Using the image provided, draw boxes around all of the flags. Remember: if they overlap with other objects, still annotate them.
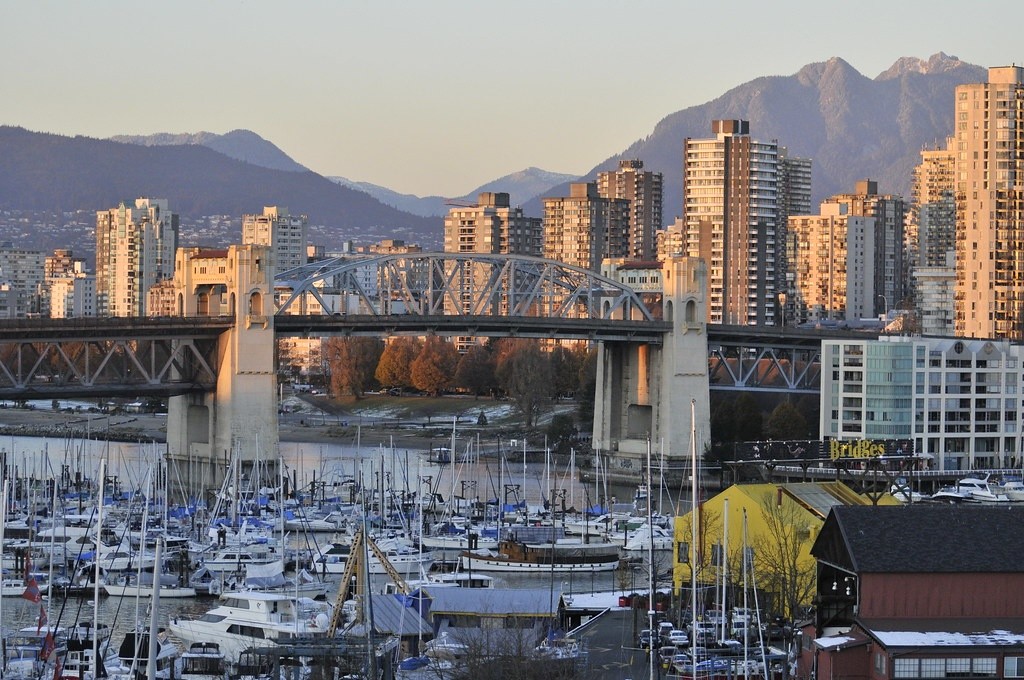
[22,578,41,603]
[22,554,32,586]
[40,631,55,660]
[37,605,48,636]
[53,657,62,680]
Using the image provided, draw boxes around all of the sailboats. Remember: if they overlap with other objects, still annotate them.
[0,413,776,680]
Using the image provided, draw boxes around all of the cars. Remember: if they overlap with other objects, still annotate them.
[633,605,755,667]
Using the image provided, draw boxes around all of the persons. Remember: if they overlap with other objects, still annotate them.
[750,437,807,460]
